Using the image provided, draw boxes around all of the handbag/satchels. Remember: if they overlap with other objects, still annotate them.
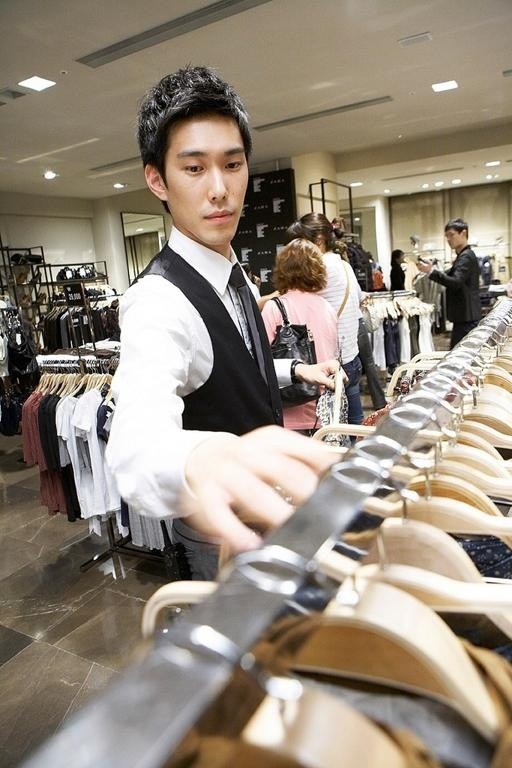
[270,296,321,408]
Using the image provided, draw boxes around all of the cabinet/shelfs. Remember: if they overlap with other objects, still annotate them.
[46,261,109,302]
[5,246,50,354]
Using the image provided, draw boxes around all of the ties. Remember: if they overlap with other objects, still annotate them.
[228,261,269,390]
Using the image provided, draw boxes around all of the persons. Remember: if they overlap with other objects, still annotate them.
[417,218,482,348]
[358,312,391,408]
[260,238,341,438]
[106,65,340,557]
[254,214,365,440]
[391,248,405,291]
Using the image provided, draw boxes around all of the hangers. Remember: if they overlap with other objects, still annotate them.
[32,355,120,401]
[48,300,73,319]
